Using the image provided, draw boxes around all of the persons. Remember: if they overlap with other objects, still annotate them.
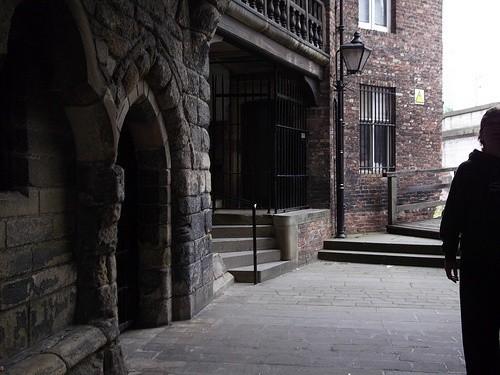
[441,108,500,373]
